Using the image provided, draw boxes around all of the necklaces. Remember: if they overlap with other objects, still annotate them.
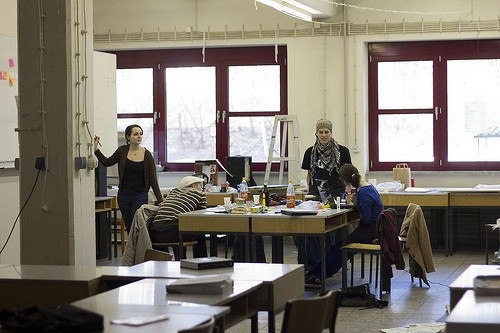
[134,153,136,155]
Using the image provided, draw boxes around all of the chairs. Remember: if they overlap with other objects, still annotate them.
[483,223,500,265]
[341,208,406,296]
[280,287,343,333]
[122,204,197,266]
[369,203,436,289]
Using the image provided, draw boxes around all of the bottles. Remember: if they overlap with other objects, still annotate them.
[261,183,269,208]
[240,177,248,199]
[286,181,295,208]
[347,187,356,204]
[245,192,253,211]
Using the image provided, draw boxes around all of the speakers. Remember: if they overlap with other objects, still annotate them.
[224,157,252,187]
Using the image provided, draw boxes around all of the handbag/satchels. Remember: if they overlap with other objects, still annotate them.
[392,163,411,187]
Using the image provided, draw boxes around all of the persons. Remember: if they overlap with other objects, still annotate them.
[301,118,352,200]
[149,176,208,258]
[94,125,163,235]
[305,163,384,288]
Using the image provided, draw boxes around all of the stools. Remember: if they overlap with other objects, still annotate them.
[205,234,228,259]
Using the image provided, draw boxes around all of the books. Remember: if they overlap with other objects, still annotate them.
[165,274,234,295]
[473,275,500,297]
[281,209,318,216]
[180,256,235,270]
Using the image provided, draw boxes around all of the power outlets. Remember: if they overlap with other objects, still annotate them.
[75,156,86,170]
[35,157,45,170]
[87,155,99,170]
[351,145,359,154]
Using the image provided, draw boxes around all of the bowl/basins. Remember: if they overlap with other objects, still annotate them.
[155,165,165,172]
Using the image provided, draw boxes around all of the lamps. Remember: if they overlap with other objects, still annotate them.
[256,0,313,22]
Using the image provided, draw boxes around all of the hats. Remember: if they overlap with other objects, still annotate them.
[179,176,205,188]
[315,118,333,132]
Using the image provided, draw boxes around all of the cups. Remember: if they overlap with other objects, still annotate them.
[249,206,263,213]
[223,197,230,204]
[235,198,244,207]
[225,201,231,208]
[253,194,260,205]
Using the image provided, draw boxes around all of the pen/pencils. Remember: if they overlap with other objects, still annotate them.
[95,135,103,147]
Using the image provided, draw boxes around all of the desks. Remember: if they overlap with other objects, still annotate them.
[0,168,500,333]
